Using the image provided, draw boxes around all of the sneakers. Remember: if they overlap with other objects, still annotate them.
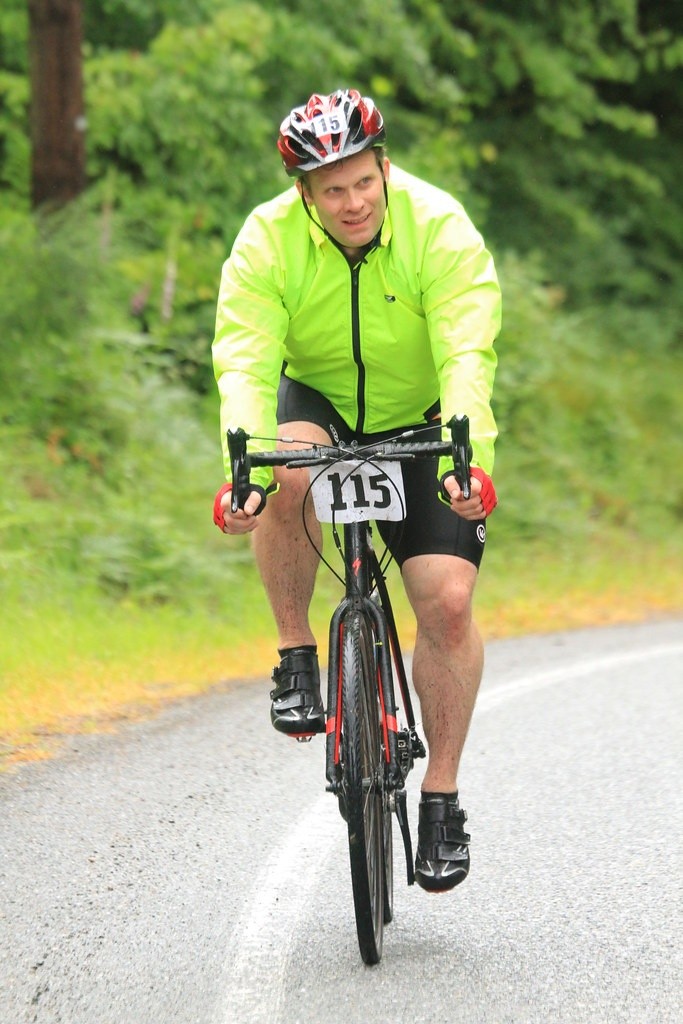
[414,792,471,891]
[269,649,326,735]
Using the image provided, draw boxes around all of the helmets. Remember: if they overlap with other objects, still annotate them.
[278,88,385,176]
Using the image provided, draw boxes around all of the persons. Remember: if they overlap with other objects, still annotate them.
[212,89,503,891]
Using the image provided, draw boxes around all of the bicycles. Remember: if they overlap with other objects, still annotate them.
[221,415,476,965]
